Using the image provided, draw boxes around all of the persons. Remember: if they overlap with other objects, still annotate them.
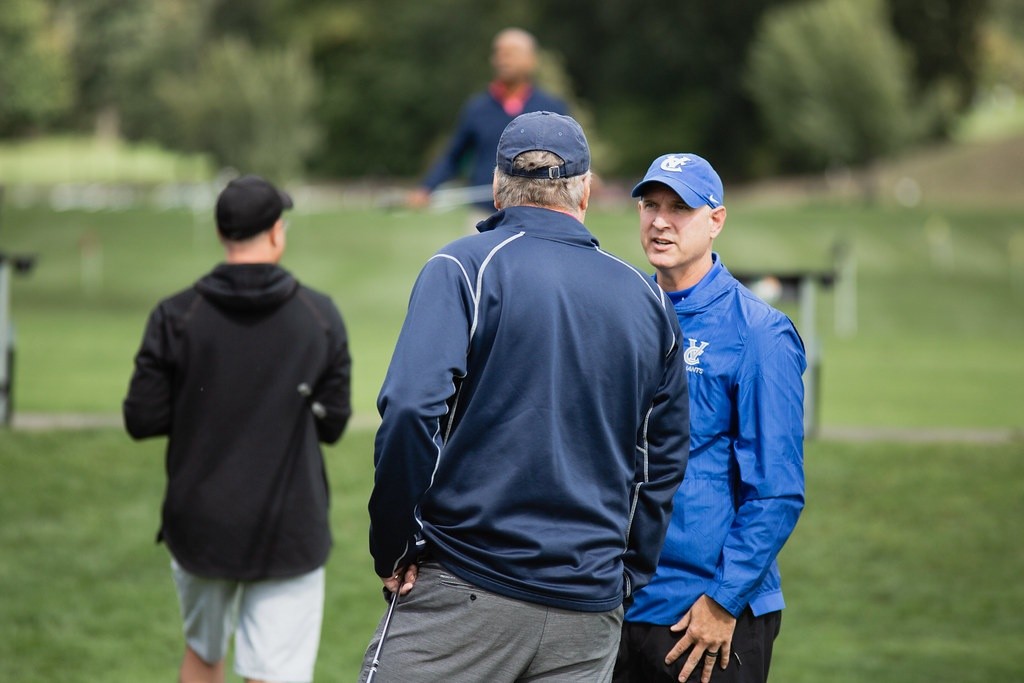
[611,152,809,683]
[122,175,352,683]
[405,27,602,235]
[361,111,689,683]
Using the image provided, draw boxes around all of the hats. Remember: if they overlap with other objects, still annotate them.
[217,175,292,241]
[630,153,723,210]
[497,109,590,179]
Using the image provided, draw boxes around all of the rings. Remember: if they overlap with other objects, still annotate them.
[707,652,718,657]
[394,575,398,578]
